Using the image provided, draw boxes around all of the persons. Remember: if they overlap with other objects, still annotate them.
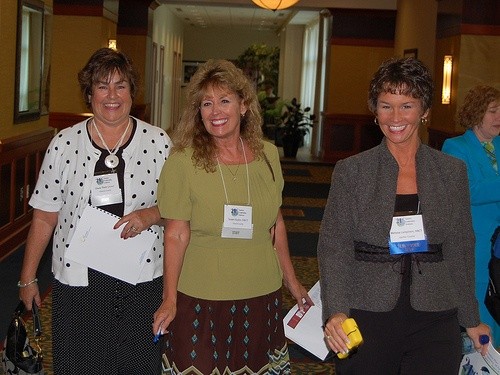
[441,85,500,357]
[152,58,315,375]
[316,56,491,375]
[18,47,176,375]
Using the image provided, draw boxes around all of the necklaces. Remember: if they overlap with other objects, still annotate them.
[92,117,131,168]
[218,149,243,184]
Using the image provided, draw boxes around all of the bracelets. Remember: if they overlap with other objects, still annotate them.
[17,278,38,288]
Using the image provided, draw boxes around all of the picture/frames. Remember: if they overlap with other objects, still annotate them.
[182,60,206,87]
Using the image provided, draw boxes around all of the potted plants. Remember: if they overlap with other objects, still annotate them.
[262,98,291,140]
[276,96,317,159]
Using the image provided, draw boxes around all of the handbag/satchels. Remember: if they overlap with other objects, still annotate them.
[484,225,500,327]
[0,299,44,375]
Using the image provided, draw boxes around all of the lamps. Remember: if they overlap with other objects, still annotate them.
[250,0,299,12]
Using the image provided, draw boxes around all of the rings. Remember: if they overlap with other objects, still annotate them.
[326,335,333,340]
[131,226,137,231]
